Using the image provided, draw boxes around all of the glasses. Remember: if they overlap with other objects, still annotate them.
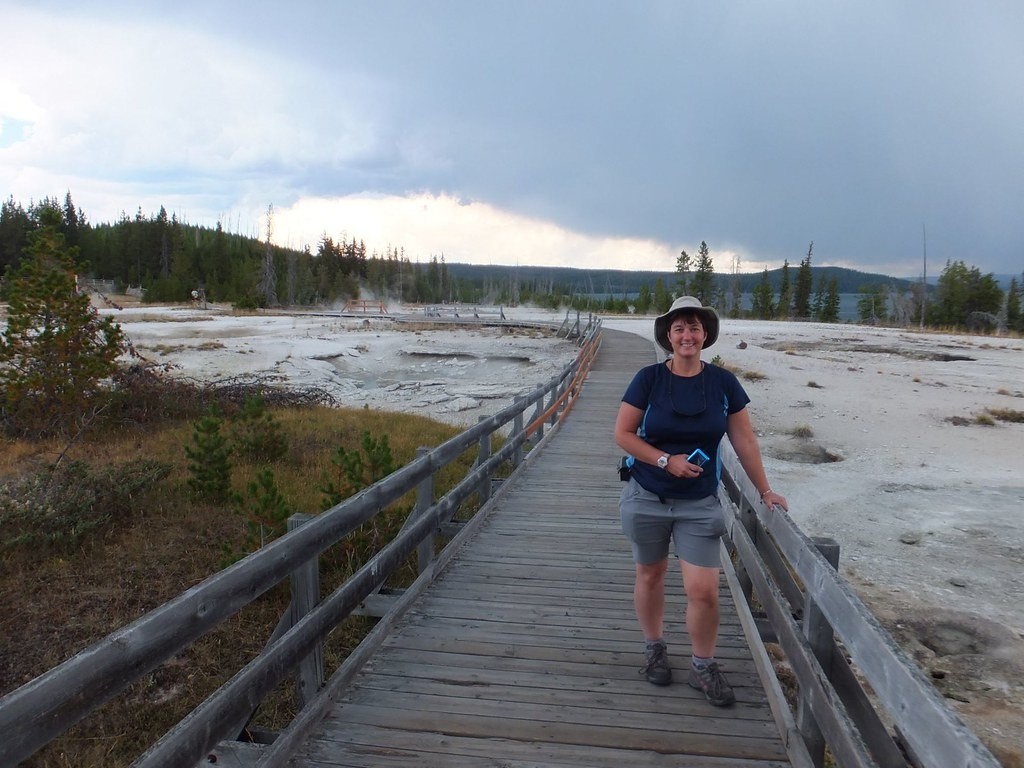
[670,388,706,416]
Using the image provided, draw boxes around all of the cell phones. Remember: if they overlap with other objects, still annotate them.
[686,448,710,473]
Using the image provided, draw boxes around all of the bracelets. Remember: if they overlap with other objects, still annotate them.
[760,489,772,499]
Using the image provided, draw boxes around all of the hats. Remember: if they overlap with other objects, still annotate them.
[653,296,720,354]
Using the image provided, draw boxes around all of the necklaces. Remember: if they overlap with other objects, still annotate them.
[670,358,703,374]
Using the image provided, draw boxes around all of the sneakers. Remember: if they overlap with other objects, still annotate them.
[688,662,735,705]
[637,642,672,685]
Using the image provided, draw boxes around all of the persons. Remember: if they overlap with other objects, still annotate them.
[614,296,788,706]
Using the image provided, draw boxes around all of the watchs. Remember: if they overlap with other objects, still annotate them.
[657,453,670,469]
